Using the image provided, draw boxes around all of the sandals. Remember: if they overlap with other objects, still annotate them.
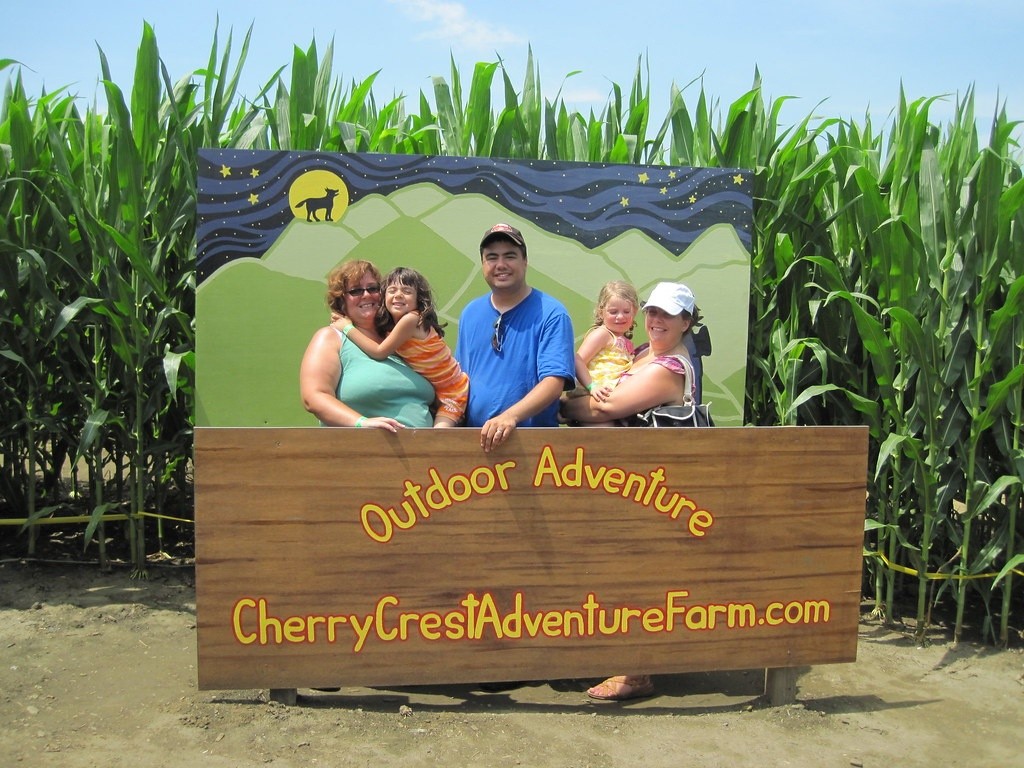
[587,674,655,700]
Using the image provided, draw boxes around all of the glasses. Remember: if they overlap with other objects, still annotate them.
[491,314,504,352]
[344,286,379,296]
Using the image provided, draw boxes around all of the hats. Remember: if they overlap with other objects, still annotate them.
[480,224,525,253]
[641,282,695,317]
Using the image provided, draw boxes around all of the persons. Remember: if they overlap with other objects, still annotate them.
[565,281,640,428]
[558,280,703,428]
[299,259,436,432]
[329,266,469,428]
[454,224,576,452]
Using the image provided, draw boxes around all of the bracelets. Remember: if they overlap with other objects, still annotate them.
[343,323,355,334]
[587,382,597,392]
[355,416,366,427]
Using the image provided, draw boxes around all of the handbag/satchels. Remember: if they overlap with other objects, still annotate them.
[632,354,715,427]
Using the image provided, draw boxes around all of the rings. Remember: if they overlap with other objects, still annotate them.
[497,430,503,433]
